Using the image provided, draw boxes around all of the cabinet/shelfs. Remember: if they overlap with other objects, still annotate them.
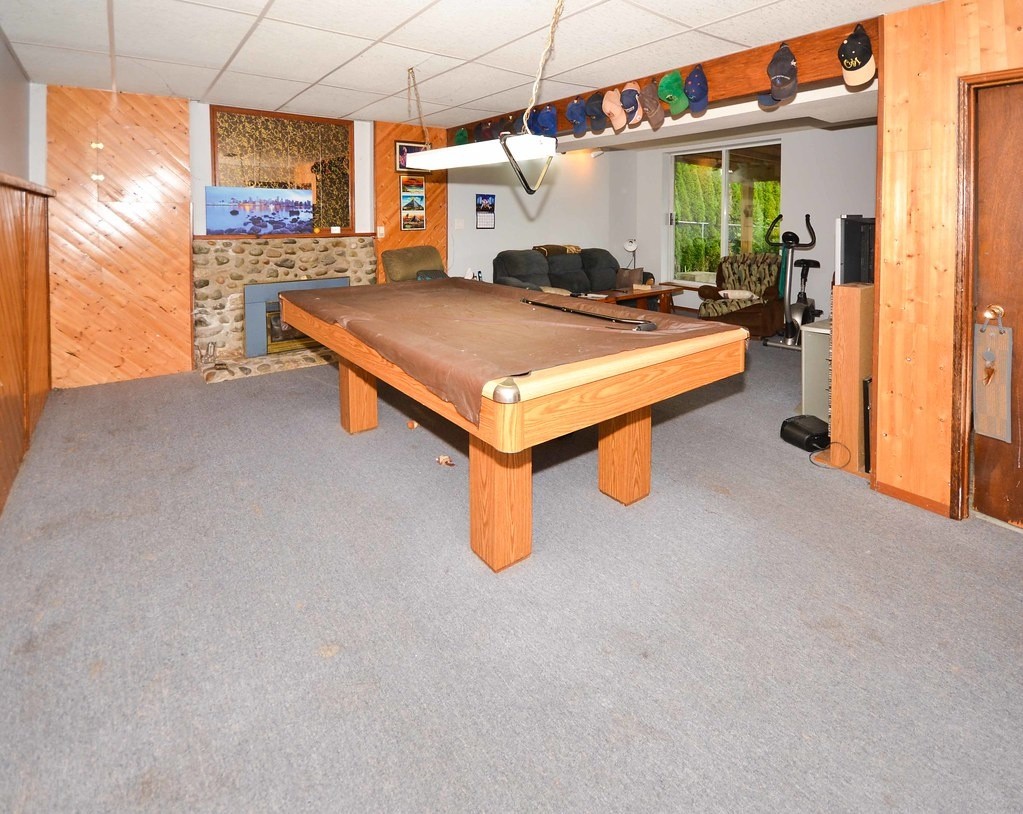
[814,281,874,478]
[800,317,832,425]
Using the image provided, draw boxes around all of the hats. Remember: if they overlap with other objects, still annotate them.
[454,128,468,144]
[767,42,797,100]
[657,70,688,115]
[838,24,875,86]
[620,80,643,125]
[585,92,605,131]
[472,104,557,143]
[758,91,779,106]
[684,64,708,113]
[602,88,627,130]
[566,97,588,138]
[638,77,665,126]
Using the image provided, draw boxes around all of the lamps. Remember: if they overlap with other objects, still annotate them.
[405,1,568,171]
[623,238,638,269]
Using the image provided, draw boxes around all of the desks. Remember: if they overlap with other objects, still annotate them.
[591,285,680,313]
[278,275,751,572]
[659,280,717,312]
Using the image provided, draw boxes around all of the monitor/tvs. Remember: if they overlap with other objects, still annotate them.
[834,218,876,285]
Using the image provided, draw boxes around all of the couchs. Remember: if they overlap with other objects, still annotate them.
[493,245,658,311]
[380,246,444,283]
[698,253,785,341]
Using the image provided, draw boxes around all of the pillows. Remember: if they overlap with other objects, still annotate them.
[539,286,572,297]
[717,289,760,301]
[616,268,645,289]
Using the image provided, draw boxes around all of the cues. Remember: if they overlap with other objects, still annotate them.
[521,298,657,332]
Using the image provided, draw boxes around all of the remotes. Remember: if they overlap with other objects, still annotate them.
[587,294,608,298]
[840,215,862,219]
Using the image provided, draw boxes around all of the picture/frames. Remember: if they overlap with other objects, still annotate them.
[394,140,432,175]
